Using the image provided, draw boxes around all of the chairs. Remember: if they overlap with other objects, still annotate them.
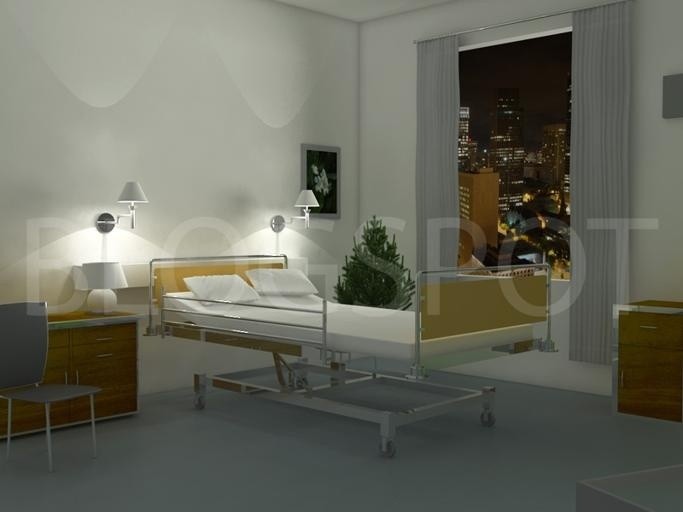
[0,301,100,473]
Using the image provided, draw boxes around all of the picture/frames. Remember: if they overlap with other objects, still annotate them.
[300,142,343,221]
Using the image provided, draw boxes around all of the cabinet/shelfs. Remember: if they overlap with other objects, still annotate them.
[616,299,683,424]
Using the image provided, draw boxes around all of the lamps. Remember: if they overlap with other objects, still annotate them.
[271,189,320,233]
[75,261,129,315]
[96,181,149,234]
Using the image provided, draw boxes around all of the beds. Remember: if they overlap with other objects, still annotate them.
[142,253,559,458]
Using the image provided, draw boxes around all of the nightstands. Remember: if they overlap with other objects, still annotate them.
[0,308,140,437]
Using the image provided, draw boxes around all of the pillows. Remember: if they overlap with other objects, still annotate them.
[182,273,260,307]
[243,268,320,297]
